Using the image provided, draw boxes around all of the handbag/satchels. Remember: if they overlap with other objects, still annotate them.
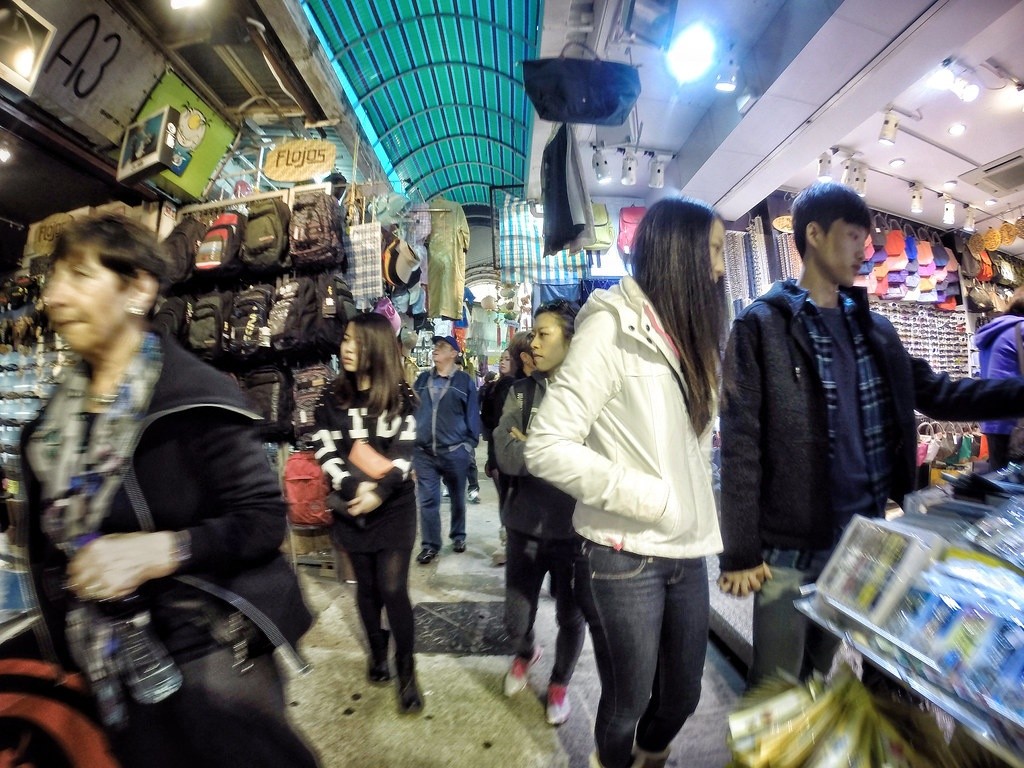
[915,421,989,466]
[522,39,642,127]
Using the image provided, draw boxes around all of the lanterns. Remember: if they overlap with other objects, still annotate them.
[481,284,530,341]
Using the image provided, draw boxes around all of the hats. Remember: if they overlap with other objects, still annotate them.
[431,335,460,352]
[373,236,427,385]
[484,371,498,382]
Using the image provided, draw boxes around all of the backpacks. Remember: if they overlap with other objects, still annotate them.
[583,200,614,251]
[617,203,649,254]
[155,180,365,533]
[961,238,1024,321]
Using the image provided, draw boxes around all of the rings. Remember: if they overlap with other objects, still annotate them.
[85,582,101,595]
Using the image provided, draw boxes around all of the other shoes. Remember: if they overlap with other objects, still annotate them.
[417,548,439,562]
[442,485,450,497]
[364,652,392,685]
[452,539,466,551]
[397,680,425,714]
[467,487,481,502]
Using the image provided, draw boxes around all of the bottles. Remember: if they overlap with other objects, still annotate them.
[73,529,183,705]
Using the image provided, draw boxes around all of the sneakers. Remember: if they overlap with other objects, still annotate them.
[503,644,545,697]
[546,682,571,725]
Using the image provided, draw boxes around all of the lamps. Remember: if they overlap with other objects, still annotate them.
[854,166,868,198]
[621,151,638,186]
[842,159,855,186]
[910,190,924,213]
[877,113,900,146]
[714,52,741,92]
[962,207,976,232]
[648,158,664,189]
[942,193,955,226]
[592,151,612,184]
[818,153,834,183]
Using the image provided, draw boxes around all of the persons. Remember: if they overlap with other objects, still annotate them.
[18,211,323,768]
[716,183,1024,707]
[442,331,537,565]
[523,197,772,767]
[974,298,1024,468]
[412,336,481,564]
[311,311,426,715]
[491,299,586,726]
